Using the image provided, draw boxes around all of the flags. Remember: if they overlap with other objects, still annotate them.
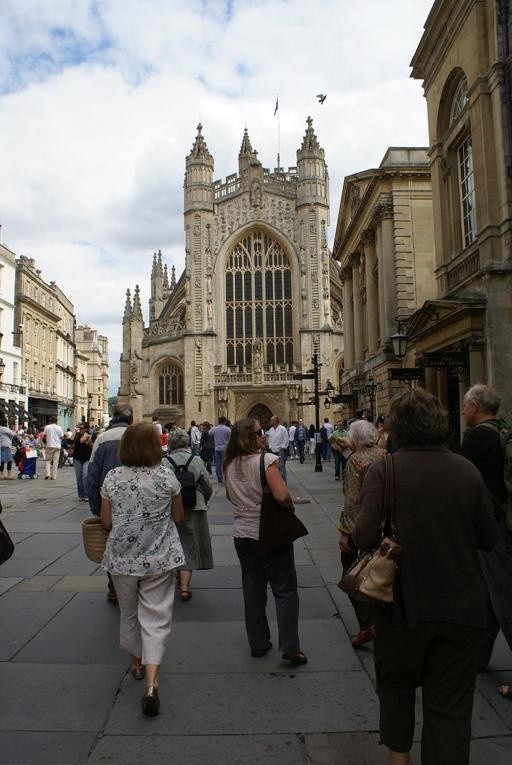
[274,97,278,114]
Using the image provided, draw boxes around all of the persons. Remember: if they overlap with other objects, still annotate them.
[340,420,389,648]
[458,383,512,702]
[352,386,498,765]
[0,414,112,500]
[151,408,389,486]
[100,421,184,716]
[222,418,308,665]
[87,408,140,601]
[161,429,213,599]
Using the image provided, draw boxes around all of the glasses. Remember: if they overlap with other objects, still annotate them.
[253,428,264,438]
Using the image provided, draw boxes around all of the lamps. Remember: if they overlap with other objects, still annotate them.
[323,398,331,409]
[326,379,336,398]
[389,316,410,357]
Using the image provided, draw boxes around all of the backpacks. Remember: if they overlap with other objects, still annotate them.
[461,423,512,531]
[166,454,197,509]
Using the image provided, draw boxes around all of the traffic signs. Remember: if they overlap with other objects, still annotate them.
[309,397,315,400]
[292,374,315,380]
[318,392,330,396]
[295,402,314,406]
[305,369,314,373]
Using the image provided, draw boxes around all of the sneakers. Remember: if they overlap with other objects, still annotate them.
[251,641,274,659]
[130,655,146,681]
[351,625,376,648]
[282,649,308,664]
[108,589,117,600]
[142,685,160,720]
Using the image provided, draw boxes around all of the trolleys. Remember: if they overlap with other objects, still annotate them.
[13,437,39,480]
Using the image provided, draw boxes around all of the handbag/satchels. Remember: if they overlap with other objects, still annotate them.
[259,451,310,542]
[81,517,113,564]
[336,451,406,609]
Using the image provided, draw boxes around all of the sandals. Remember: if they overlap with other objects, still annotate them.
[179,587,192,602]
